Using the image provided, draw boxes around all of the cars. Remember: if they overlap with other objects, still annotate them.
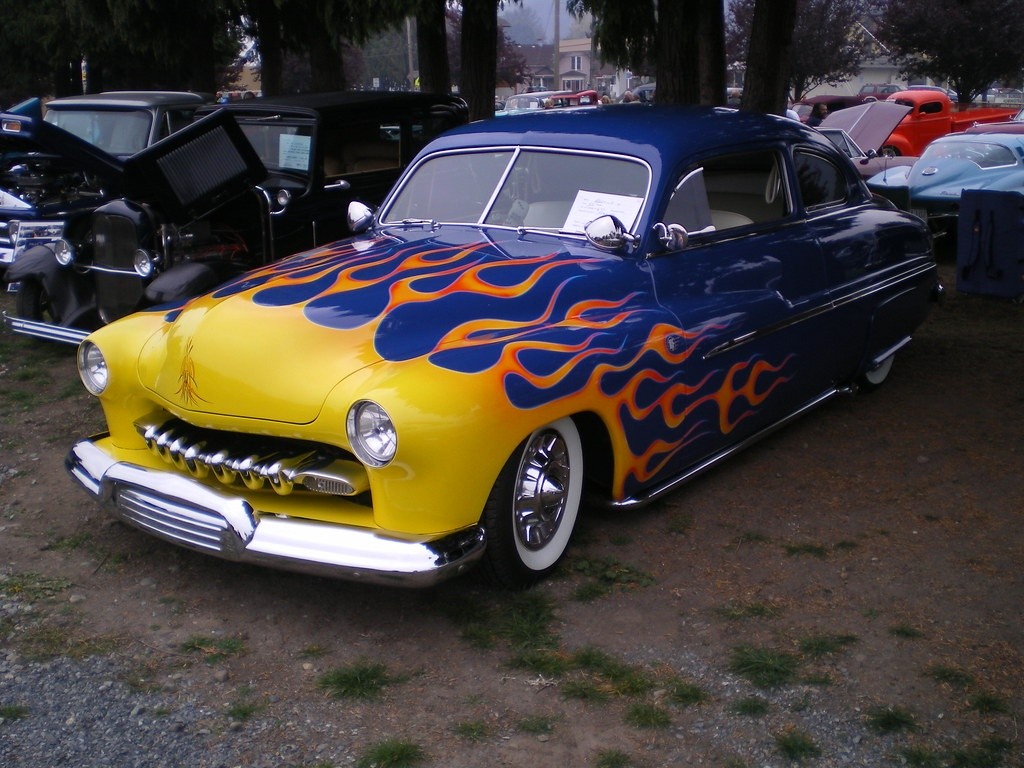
[818,83,1024,243]
[493,88,602,112]
[0,89,470,345]
[615,83,655,104]
[67,105,956,587]
[41,90,212,159]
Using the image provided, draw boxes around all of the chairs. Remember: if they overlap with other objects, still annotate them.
[353,158,397,173]
[710,210,753,232]
[523,200,573,227]
[323,156,343,176]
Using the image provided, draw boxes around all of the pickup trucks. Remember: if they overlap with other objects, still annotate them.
[876,91,1021,156]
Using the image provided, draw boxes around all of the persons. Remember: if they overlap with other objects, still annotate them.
[601,90,642,105]
[786,104,828,128]
[542,98,557,111]
[243,91,255,99]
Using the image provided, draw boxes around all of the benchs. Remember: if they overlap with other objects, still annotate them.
[331,141,400,174]
[585,171,783,224]
